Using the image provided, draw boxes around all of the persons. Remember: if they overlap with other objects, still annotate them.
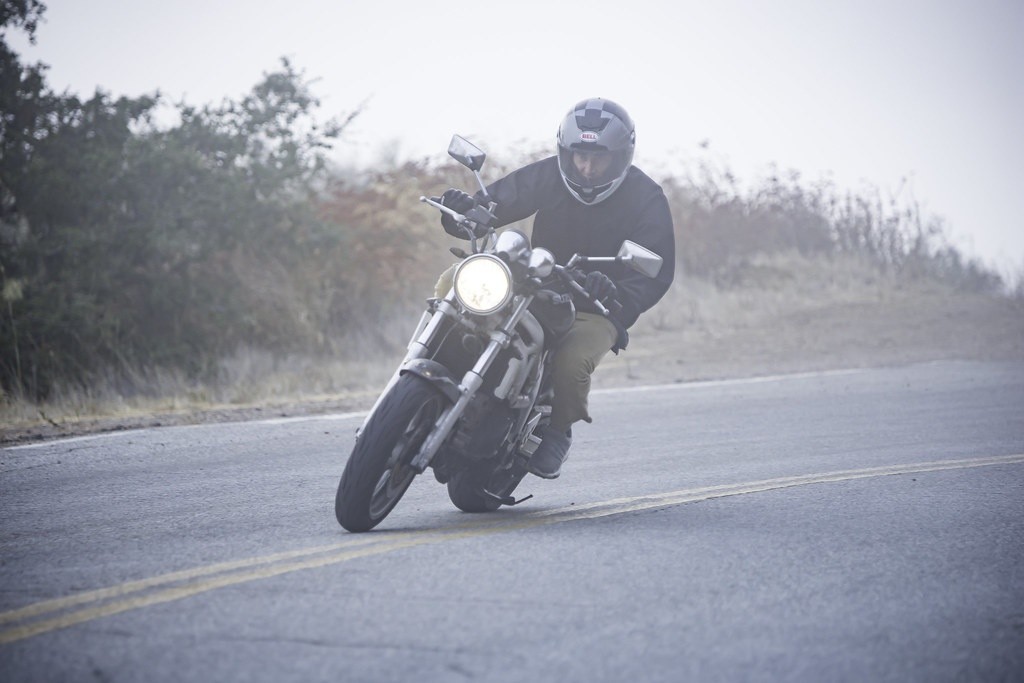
[437,97,676,478]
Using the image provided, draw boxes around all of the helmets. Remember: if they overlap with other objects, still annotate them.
[556,96,636,206]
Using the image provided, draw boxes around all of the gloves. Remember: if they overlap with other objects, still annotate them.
[586,270,617,308]
[440,188,473,218]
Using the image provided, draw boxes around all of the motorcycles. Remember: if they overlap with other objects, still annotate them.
[335,133,664,533]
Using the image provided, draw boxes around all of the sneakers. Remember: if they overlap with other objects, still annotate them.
[529,426,574,479]
